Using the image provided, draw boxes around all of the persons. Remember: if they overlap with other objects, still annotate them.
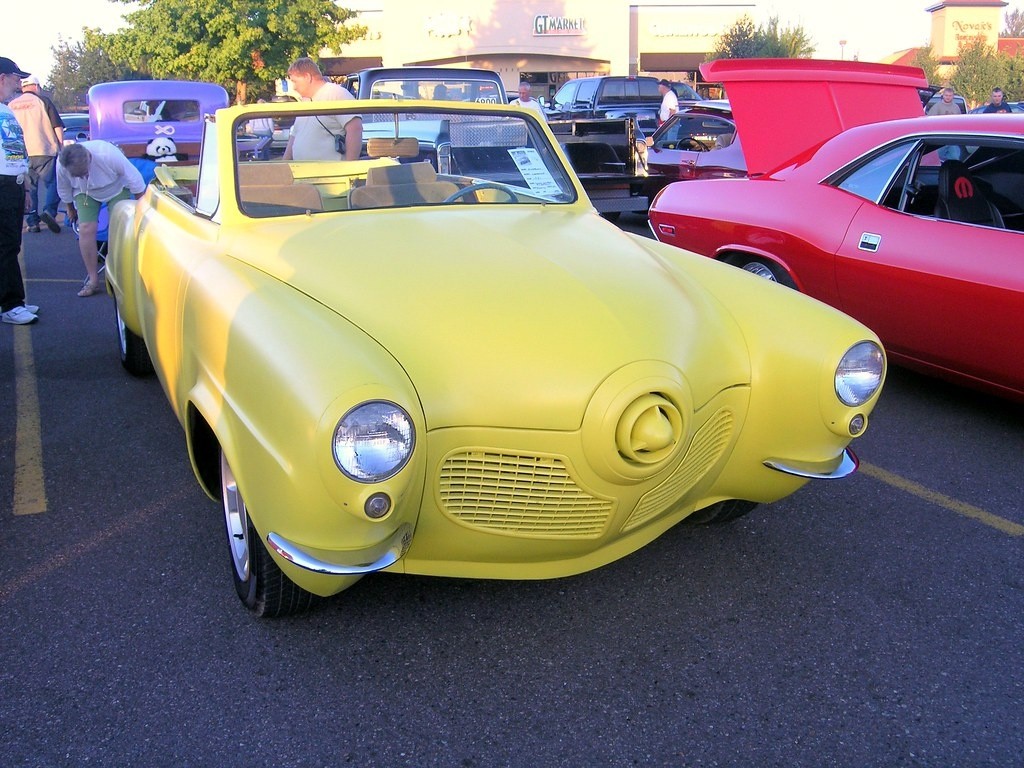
[249,98,274,160]
[657,79,680,142]
[7,76,65,231]
[0,56,40,325]
[56,140,147,296]
[928,88,961,115]
[282,58,363,161]
[983,88,1012,113]
[510,81,544,119]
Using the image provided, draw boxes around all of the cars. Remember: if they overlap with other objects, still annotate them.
[78,80,229,241]
[667,82,705,110]
[967,102,1024,114]
[60,116,90,147]
[649,58,1024,399]
[646,99,748,198]
[104,98,887,618]
[269,120,293,148]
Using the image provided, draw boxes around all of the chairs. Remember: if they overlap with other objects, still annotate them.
[934,159,1004,230]
[60,157,159,285]
[710,133,732,151]
[348,162,462,209]
[238,162,324,216]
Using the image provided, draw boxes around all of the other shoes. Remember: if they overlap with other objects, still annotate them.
[40,210,61,233]
[26,226,41,233]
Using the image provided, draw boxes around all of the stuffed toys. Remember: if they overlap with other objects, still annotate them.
[146,137,186,162]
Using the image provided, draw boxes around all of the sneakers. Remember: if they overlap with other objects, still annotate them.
[77,280,100,297]
[23,304,40,314]
[2,306,38,325]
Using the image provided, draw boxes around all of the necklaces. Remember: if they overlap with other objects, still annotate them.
[78,174,90,206]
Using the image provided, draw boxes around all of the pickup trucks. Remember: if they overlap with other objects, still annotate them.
[536,76,662,138]
[338,66,648,212]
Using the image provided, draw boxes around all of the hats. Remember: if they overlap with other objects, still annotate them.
[0,56,32,79]
[656,79,671,88]
[21,76,39,86]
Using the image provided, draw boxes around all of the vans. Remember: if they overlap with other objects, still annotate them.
[923,94,968,116]
